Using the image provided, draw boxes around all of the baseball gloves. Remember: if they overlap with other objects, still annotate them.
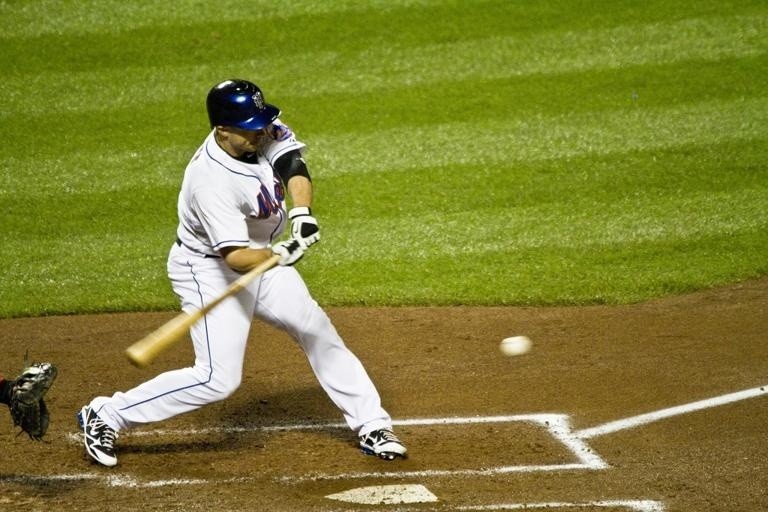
[8,360,57,444]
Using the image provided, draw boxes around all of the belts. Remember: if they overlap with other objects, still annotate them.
[173,234,223,262]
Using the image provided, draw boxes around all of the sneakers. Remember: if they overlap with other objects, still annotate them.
[71,403,123,470]
[354,430,410,463]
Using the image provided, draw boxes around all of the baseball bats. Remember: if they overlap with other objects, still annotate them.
[124,254,280,369]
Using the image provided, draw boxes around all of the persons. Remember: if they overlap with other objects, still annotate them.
[75,78,409,468]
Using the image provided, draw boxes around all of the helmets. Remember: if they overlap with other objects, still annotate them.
[204,76,282,134]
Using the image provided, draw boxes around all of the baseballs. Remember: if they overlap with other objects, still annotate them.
[501,335,532,359]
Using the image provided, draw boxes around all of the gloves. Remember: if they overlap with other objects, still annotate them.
[270,204,325,270]
